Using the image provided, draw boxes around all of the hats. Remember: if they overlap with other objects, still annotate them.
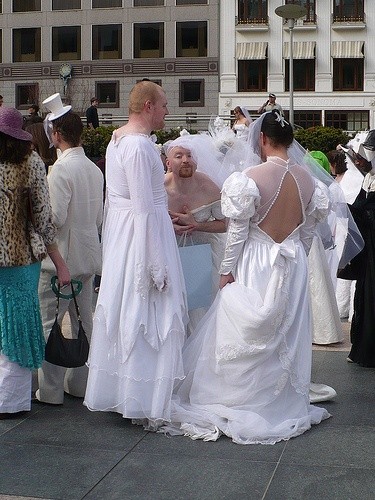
[27,104,39,113]
[41,92,72,121]
[345,130,375,173]
[0,105,33,140]
[304,150,331,178]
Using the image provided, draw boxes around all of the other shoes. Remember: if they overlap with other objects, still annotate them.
[31,391,63,406]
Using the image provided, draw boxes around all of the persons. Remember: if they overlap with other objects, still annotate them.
[257,94,284,113]
[86,98,99,130]
[93,141,109,292]
[82,81,190,420]
[325,150,356,318]
[0,107,70,419]
[230,106,253,136]
[301,150,344,344]
[163,134,230,341]
[23,103,44,130]
[333,129,375,368]
[28,110,105,408]
[24,123,55,176]
[216,109,319,445]
[0,95,3,107]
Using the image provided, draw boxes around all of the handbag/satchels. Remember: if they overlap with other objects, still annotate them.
[45,283,89,368]
[178,232,213,310]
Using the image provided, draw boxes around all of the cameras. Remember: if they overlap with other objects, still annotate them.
[93,275,101,293]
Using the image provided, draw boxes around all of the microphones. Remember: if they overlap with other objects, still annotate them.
[265,100,269,106]
